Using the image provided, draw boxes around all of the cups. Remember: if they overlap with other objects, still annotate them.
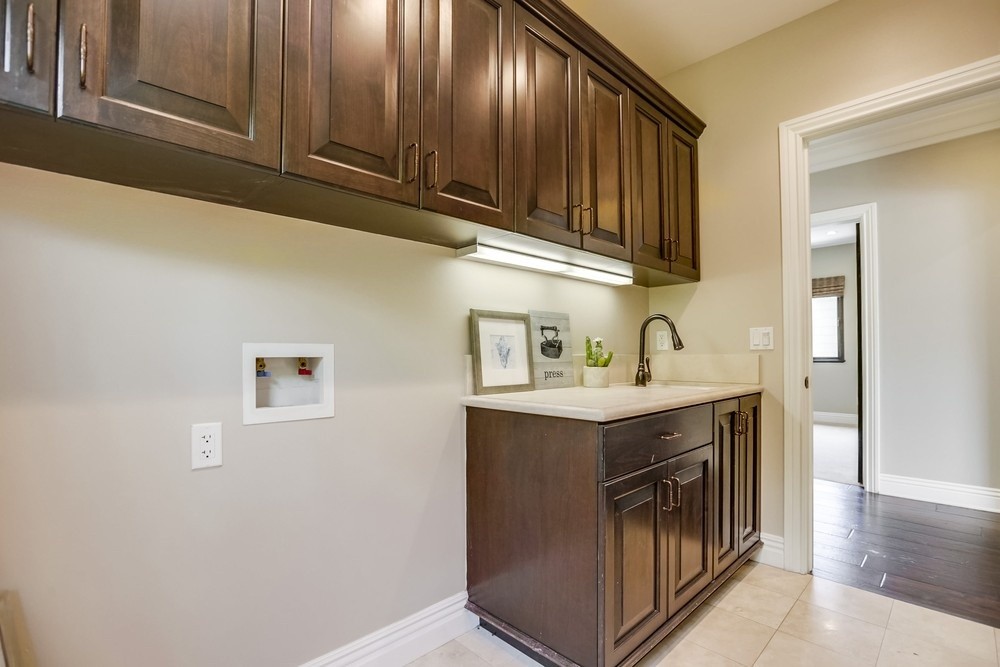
[583,365,611,388]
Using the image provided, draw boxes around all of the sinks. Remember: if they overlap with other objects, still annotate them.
[636,385,719,392]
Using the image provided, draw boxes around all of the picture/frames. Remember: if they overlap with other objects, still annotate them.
[470,308,534,396]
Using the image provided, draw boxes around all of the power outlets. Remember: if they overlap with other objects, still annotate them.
[191,423,222,469]
[657,331,667,350]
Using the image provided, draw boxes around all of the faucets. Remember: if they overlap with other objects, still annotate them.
[635,314,684,386]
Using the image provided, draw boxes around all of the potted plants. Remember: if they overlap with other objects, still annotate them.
[584,337,613,388]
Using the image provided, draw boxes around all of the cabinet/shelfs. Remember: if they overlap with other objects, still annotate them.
[713,393,761,580]
[515,2,632,263]
[628,88,701,289]
[0,0,284,208]
[467,404,713,667]
[280,0,515,250]
